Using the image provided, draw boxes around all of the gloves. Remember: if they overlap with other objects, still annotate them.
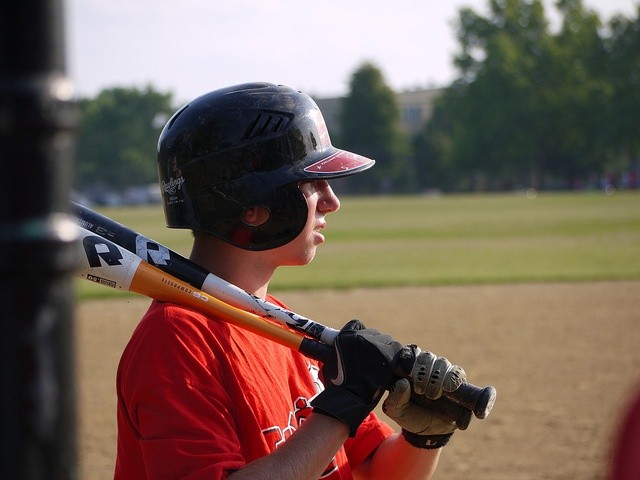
[382,343,467,451]
[308,320,400,437]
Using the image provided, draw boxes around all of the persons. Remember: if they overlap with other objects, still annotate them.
[113,82,468,480]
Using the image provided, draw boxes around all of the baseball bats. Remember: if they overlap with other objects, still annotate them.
[70,201,496,419]
[68,222,472,430]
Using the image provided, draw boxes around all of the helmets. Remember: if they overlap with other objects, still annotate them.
[157,80,375,251]
